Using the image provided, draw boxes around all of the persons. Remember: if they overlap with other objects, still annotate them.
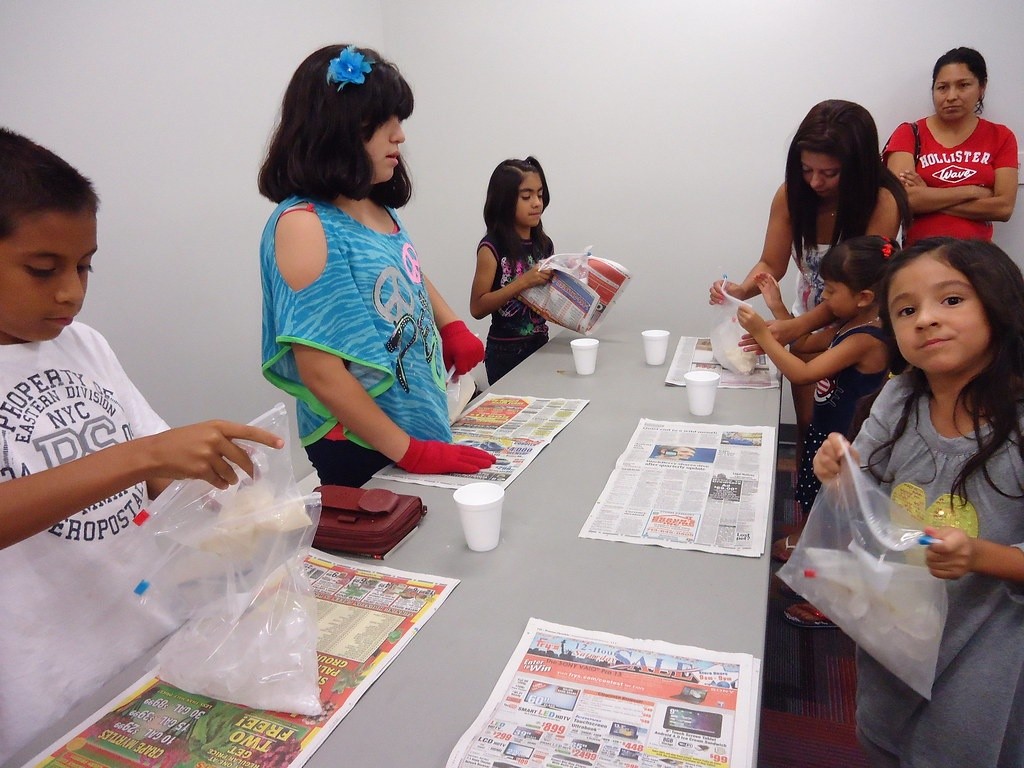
[258,43,496,487]
[470,156,554,387]
[886,48,1018,251]
[0,129,285,768]
[812,236,1024,768]
[709,100,902,627]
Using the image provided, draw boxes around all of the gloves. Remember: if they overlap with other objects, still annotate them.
[439,321,484,384]
[394,437,497,475]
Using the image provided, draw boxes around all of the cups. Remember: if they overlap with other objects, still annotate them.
[683,371,720,417]
[570,338,600,375]
[641,330,670,365]
[453,482,505,552]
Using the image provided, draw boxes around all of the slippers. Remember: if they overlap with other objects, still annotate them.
[772,536,796,562]
[782,603,839,628]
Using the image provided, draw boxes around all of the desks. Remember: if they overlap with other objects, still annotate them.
[1,328,790,768]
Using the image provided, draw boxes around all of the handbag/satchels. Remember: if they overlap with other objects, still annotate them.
[311,485,428,556]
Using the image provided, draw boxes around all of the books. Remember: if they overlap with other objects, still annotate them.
[314,525,419,561]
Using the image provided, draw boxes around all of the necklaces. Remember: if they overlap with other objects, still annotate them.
[828,317,880,349]
